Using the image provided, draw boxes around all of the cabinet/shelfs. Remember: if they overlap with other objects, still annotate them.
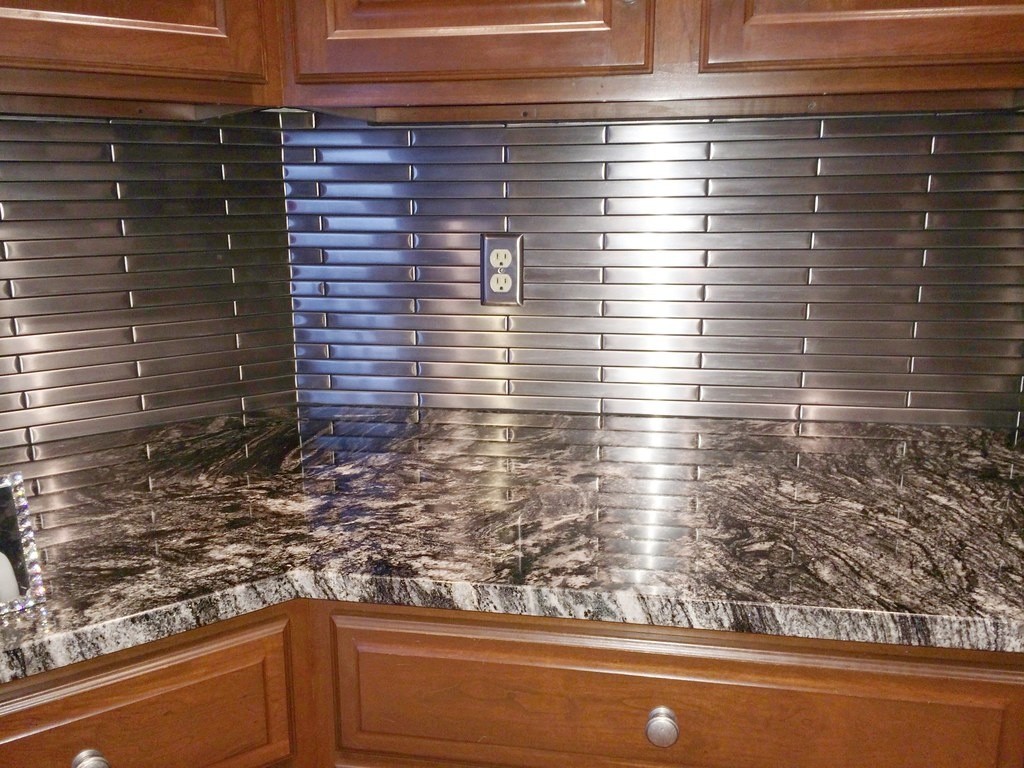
[0,0,1024,124]
[0,598,1023,768]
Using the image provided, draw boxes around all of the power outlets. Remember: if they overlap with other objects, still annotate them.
[480,233,523,307]
